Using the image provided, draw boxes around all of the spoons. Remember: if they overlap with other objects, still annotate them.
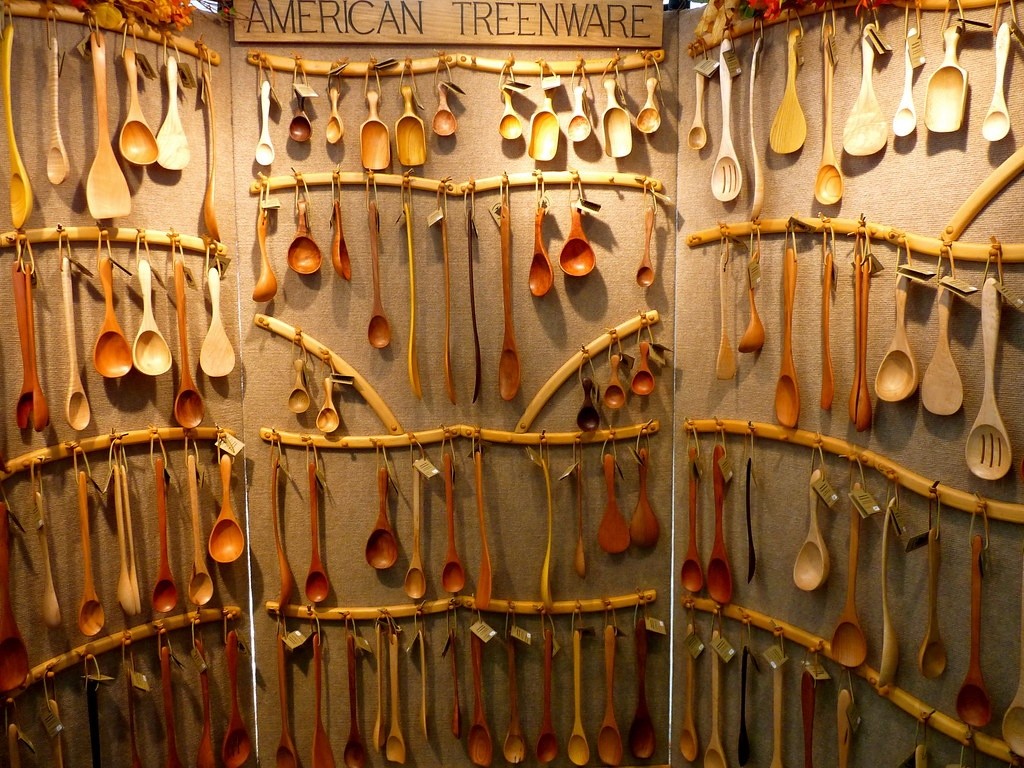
[0,6,1019,765]
